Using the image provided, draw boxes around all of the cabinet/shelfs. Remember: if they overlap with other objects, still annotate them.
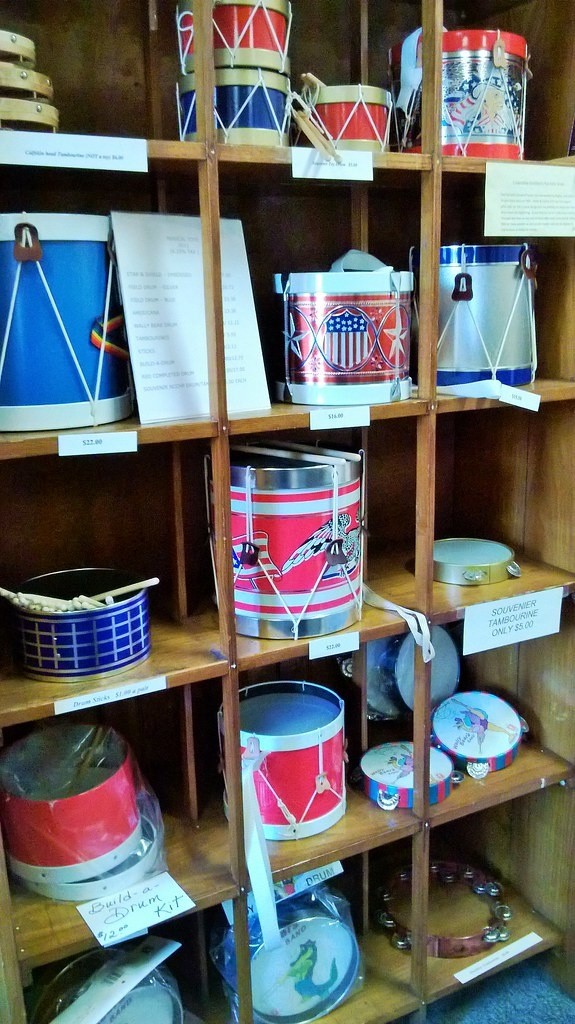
[0,2,573,1024]
